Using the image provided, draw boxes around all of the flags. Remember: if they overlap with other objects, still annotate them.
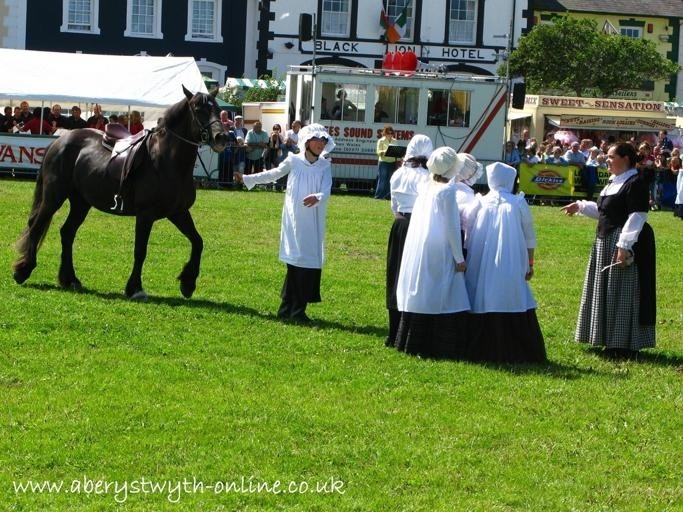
[379,8,387,30]
[383,8,406,43]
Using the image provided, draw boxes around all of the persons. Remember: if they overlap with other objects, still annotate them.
[375,126,396,200]
[232,123,335,322]
[320,85,470,126]
[559,143,655,354]
[464,161,547,365]
[503,127,682,219]
[447,153,482,261]
[0,100,299,193]
[385,133,431,347]
[396,146,472,357]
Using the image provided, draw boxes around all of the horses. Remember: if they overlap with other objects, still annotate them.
[11,83,229,304]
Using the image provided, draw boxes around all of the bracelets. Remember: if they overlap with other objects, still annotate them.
[528,259,532,266]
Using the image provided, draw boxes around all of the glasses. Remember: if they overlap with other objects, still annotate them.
[274,128,279,130]
[387,133,393,134]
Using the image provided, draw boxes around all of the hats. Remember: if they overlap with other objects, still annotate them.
[455,153,483,186]
[572,142,579,146]
[298,123,336,157]
[588,146,600,152]
[552,146,563,152]
[235,115,244,120]
[426,147,465,179]
